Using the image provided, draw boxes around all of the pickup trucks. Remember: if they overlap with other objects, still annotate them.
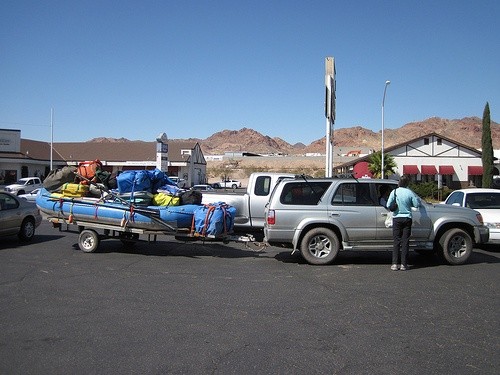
[169,176,186,188]
[4,177,45,194]
[201,173,304,227]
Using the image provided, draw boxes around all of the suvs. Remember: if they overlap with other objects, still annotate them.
[265,179,490,264]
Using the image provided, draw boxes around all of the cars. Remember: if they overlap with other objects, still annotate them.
[0,191,42,243]
[17,188,41,203]
[438,187,500,247]
[189,184,214,192]
[212,179,241,189]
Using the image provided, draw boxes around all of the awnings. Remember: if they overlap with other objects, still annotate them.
[403,165,484,175]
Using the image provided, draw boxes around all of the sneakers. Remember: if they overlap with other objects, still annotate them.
[400,264,411,270]
[391,264,398,271]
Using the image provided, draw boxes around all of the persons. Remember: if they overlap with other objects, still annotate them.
[466,195,481,208]
[378,175,421,271]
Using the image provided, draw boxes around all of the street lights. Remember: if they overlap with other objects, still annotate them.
[380,80,393,178]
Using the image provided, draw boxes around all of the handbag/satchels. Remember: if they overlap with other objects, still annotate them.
[389,188,398,212]
